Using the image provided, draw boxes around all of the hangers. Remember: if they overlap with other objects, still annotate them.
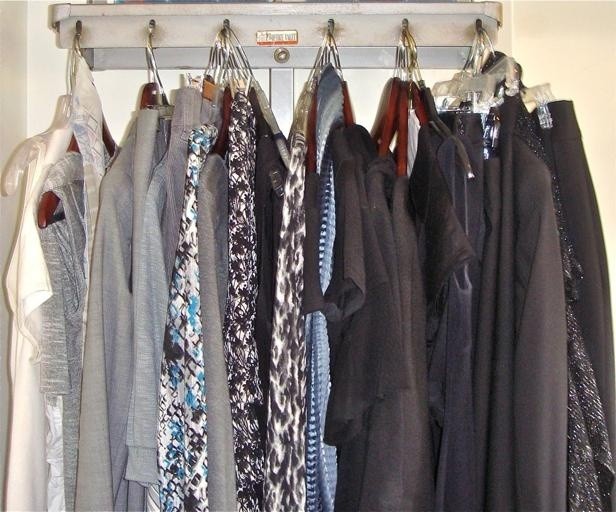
[0,28,556,229]
